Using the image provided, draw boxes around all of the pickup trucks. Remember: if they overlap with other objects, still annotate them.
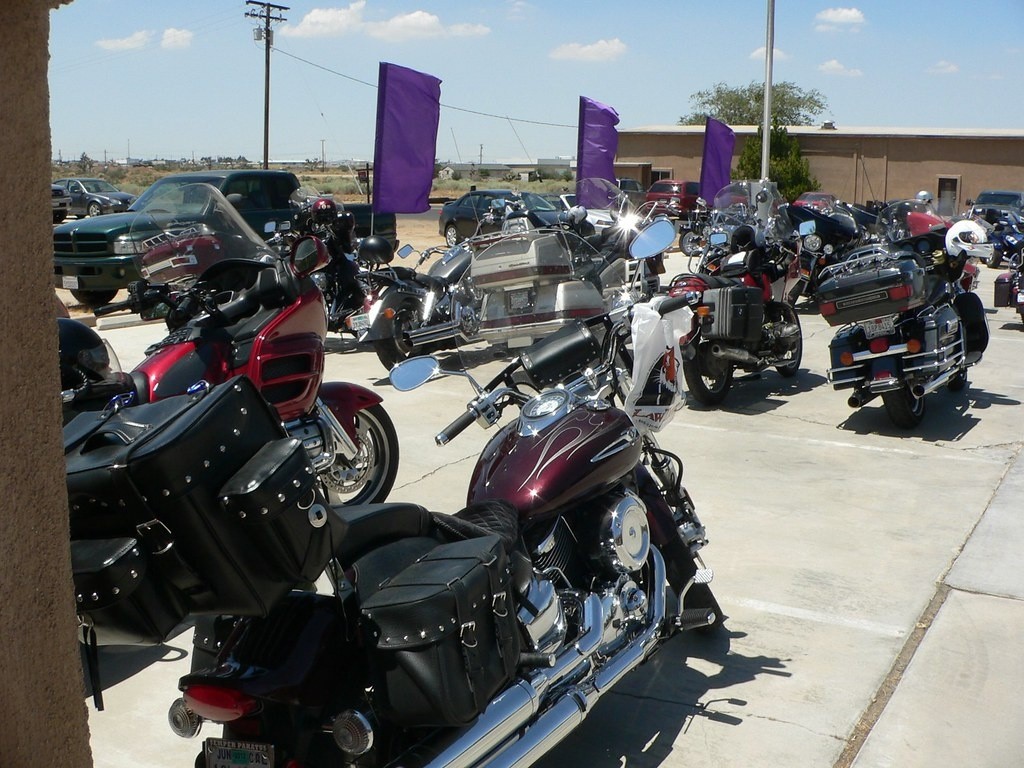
[55,167,397,306]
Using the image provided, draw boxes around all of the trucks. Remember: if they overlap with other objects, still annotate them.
[611,178,647,207]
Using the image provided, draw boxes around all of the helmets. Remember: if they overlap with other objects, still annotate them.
[311,198,336,223]
[946,219,994,260]
[918,190,933,206]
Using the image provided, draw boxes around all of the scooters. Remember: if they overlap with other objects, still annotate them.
[60,185,398,514]
[684,190,1024,324]
[218,187,683,359]
[816,195,997,422]
[61,218,731,767]
[660,183,819,410]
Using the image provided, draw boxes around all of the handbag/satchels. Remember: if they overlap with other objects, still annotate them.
[627,296,692,431]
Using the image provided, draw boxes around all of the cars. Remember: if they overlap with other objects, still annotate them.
[52,175,136,218]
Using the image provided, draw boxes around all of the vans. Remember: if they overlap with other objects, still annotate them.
[645,178,751,220]
